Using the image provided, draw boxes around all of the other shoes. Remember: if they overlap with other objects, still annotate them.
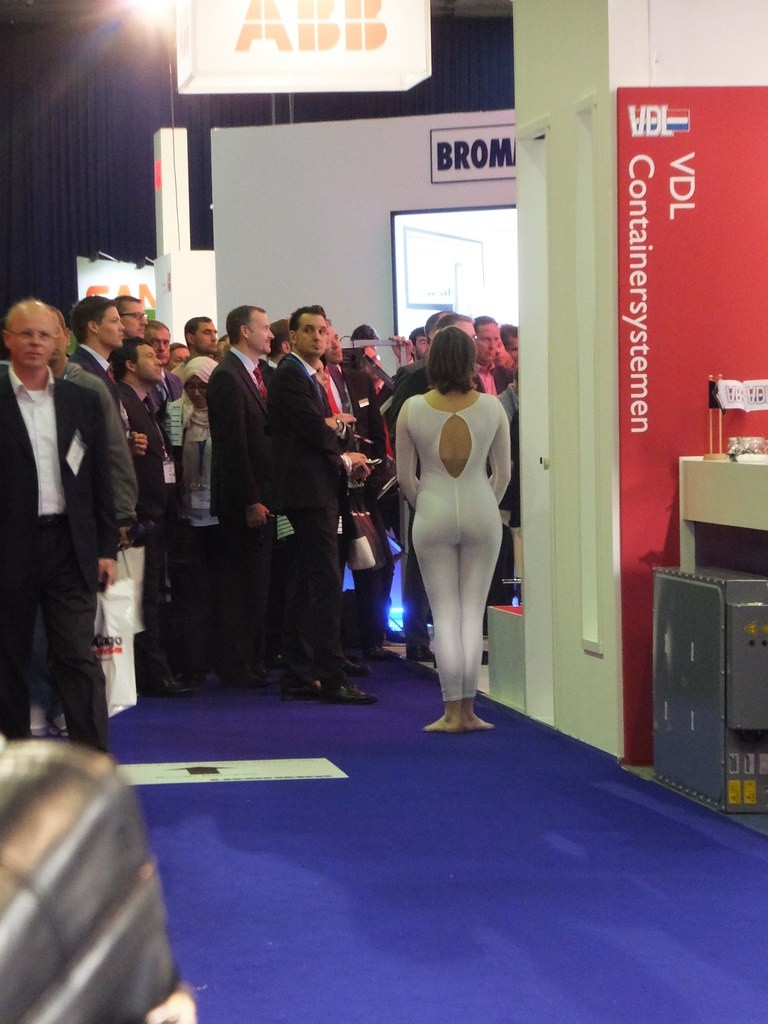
[48,714,70,737]
[369,645,387,662]
[221,672,267,690]
[271,650,296,667]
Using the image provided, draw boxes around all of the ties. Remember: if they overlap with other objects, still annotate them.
[330,365,353,430]
[254,368,267,401]
[107,368,116,385]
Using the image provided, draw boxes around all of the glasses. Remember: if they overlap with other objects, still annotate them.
[119,312,148,320]
[8,330,58,342]
[184,382,208,395]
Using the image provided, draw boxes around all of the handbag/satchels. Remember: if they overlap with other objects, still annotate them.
[344,488,385,571]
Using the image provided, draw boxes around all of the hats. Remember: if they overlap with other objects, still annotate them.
[182,356,219,386]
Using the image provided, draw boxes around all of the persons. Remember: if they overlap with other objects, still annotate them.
[0,299,118,751]
[68,295,148,457]
[206,304,276,688]
[143,318,182,404]
[30,307,139,737]
[110,335,198,697]
[164,343,190,371]
[258,318,292,388]
[167,355,219,687]
[316,311,520,677]
[394,325,512,733]
[185,316,229,351]
[268,305,376,705]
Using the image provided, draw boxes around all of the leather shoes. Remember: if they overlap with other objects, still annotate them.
[320,678,378,705]
[142,675,199,696]
[281,682,319,701]
[407,646,435,661]
[343,660,371,676]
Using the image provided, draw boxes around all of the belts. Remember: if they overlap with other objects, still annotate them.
[38,515,68,530]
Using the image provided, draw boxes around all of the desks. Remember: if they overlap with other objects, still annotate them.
[679,455,768,568]
